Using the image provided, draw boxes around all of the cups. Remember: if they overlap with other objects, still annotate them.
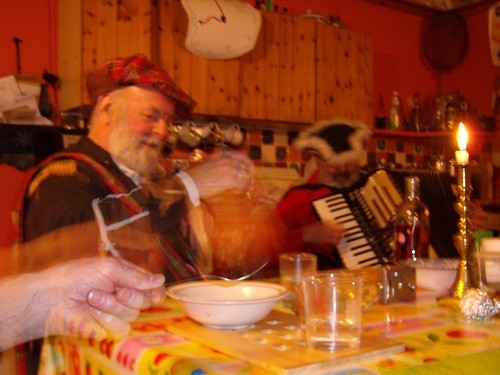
[280,252,317,323]
[166,120,243,148]
[303,272,363,350]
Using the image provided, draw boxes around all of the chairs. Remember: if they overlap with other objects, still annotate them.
[183,166,304,280]
[0,163,38,375]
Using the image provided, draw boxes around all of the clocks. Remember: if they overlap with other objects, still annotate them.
[180,0,263,62]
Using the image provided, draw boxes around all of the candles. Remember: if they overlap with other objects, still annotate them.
[455,121,469,165]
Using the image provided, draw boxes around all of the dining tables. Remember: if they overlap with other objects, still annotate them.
[36,262,499,375]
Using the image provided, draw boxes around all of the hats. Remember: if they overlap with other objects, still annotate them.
[85,54,197,120]
[297,120,373,168]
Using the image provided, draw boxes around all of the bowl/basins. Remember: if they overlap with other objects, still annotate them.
[165,280,291,330]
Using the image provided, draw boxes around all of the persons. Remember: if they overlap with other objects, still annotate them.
[254,118,436,275]
[21,55,217,374]
[1,256,167,355]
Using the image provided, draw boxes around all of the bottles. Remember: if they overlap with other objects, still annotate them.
[376,95,386,128]
[390,91,404,131]
[396,176,430,263]
[411,92,425,133]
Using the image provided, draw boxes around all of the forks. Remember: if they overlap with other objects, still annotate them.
[165,258,270,286]
